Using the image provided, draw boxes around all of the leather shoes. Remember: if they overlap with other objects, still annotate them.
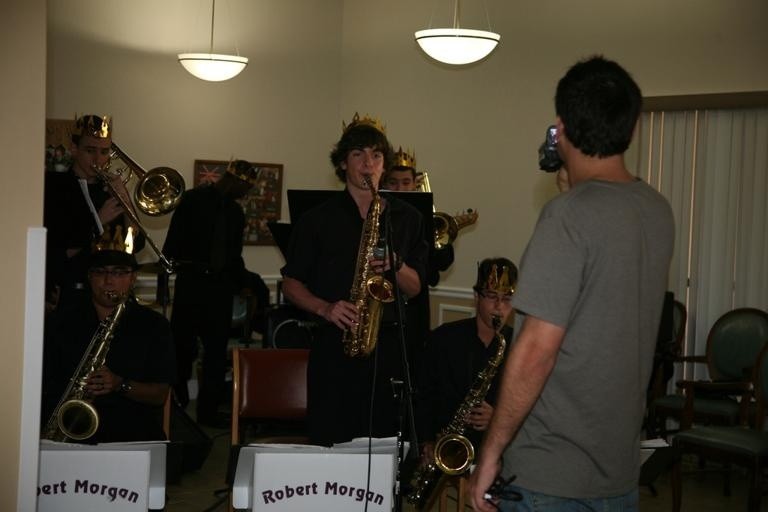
[198,411,242,428]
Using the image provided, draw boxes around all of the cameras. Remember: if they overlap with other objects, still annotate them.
[538,124,563,172]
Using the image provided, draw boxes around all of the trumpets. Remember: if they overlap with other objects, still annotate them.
[414,170,458,253]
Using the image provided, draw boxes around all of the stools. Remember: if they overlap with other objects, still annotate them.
[437,469,472,512]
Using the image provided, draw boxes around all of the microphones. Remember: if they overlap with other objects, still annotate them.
[374,224,389,259]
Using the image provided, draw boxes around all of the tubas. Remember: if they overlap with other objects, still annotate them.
[90,143,186,275]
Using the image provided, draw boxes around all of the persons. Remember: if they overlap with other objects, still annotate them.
[281,110,430,443]
[42,226,206,498]
[468,57,706,511]
[49,113,146,315]
[388,145,455,287]
[171,161,262,426]
[413,258,521,473]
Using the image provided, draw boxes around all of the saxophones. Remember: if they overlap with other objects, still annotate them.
[404,317,507,505]
[42,291,128,443]
[342,175,396,359]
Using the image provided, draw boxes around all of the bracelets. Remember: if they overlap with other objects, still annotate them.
[384,256,404,279]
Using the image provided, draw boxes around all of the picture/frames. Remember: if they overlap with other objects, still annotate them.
[192,158,284,248]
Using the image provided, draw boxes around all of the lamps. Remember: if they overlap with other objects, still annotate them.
[413,1,503,67]
[174,1,251,84]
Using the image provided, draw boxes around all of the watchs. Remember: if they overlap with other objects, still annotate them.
[117,378,132,395]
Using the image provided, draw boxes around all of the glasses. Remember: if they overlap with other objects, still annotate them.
[87,268,134,280]
[473,286,513,304]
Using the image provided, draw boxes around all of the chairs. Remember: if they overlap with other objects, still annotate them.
[161,383,176,440]
[227,345,309,512]
[667,338,768,511]
[645,306,768,488]
[636,299,687,485]
